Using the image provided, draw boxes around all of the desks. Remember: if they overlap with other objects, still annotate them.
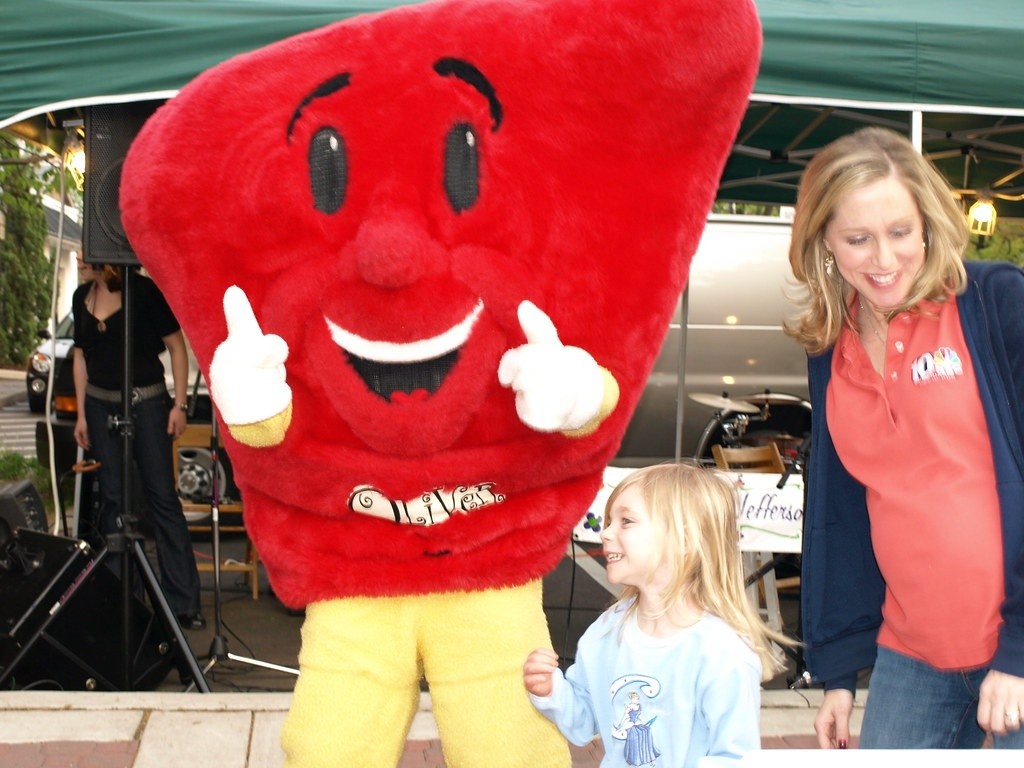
[568,465,801,685]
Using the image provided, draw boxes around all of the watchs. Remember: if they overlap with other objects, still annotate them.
[175,402,188,411]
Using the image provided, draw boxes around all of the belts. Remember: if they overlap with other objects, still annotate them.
[84,382,166,406]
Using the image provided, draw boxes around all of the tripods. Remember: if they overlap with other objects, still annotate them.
[0,257,303,693]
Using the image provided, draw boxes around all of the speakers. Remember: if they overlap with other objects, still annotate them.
[83,97,167,265]
[0,480,180,694]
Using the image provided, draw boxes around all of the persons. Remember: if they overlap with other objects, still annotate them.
[72,250,207,632]
[802,128,1024,749]
[523,463,763,767]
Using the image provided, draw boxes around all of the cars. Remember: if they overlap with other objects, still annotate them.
[25,310,75,414]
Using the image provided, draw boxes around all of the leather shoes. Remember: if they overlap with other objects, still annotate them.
[177,610,206,630]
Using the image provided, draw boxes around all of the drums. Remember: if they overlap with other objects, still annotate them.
[691,390,812,587]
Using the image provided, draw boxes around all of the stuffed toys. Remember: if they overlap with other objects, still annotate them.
[121,0,760,768]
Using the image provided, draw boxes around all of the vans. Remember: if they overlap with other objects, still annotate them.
[0,95,1024,475]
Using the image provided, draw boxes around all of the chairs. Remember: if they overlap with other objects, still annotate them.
[172,422,259,598]
[712,440,787,619]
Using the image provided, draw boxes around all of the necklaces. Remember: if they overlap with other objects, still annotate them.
[859,296,886,347]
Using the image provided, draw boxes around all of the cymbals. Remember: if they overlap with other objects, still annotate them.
[688,392,761,413]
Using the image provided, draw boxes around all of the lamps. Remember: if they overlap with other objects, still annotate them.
[961,147,999,250]
